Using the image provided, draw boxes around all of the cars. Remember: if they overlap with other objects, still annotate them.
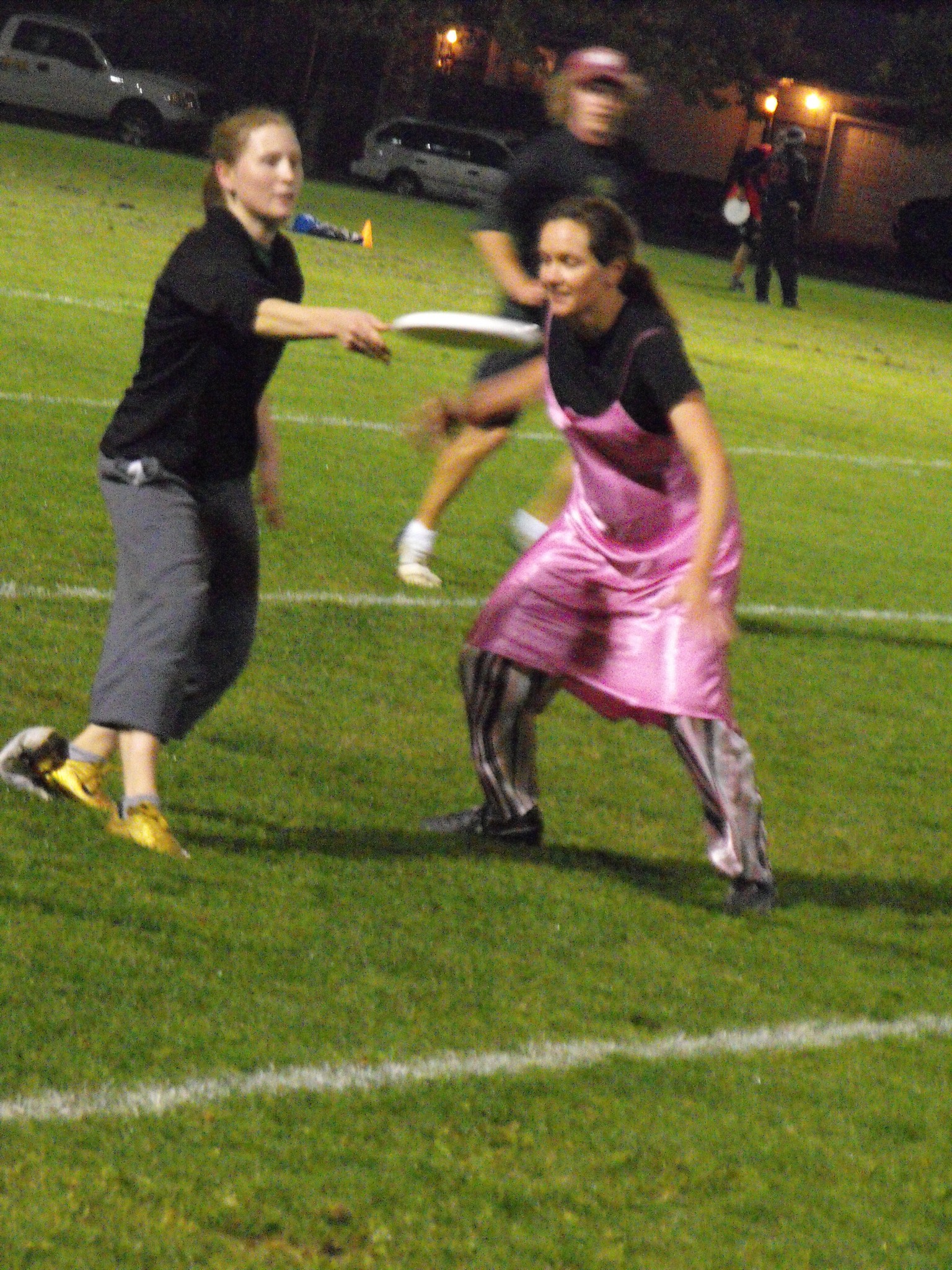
[0,14,201,143]
[346,117,514,208]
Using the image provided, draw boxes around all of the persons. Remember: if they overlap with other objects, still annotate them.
[391,191,778,919]
[397,44,671,591]
[723,124,812,312]
[0,108,393,864]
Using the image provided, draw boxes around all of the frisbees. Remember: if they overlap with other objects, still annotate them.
[724,198,751,225]
[394,308,541,353]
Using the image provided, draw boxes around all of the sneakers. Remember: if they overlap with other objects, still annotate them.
[107,804,181,861]
[419,808,545,849]
[722,877,769,921]
[36,750,112,812]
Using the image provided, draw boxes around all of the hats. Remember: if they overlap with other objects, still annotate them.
[564,45,631,90]
[786,127,805,145]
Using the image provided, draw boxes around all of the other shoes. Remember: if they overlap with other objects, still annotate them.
[395,550,437,589]
[728,276,747,291]
[508,519,544,547]
